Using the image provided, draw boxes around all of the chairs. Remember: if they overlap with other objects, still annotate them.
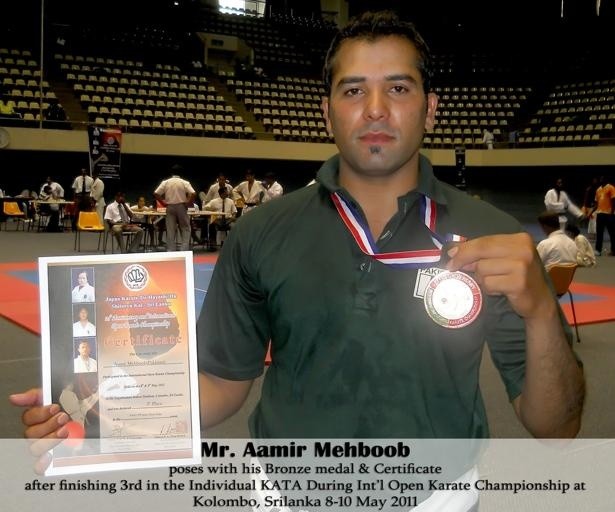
[225,74,332,143]
[55,51,257,139]
[103,205,146,253]
[75,211,106,254]
[420,87,531,146]
[130,209,153,248]
[3,201,25,231]
[204,2,336,67]
[234,198,243,218]
[545,264,581,344]
[519,79,614,147]
[0,48,69,122]
[209,209,228,247]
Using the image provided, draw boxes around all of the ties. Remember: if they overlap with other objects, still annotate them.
[82,176,85,195]
[222,199,225,224]
[118,205,126,225]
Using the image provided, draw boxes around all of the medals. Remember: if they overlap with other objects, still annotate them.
[425,273,483,330]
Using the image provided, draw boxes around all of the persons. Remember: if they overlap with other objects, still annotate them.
[536,175,615,297]
[59,379,99,426]
[73,307,95,337]
[71,270,94,303]
[74,341,97,373]
[0,163,282,254]
[8,11,586,512]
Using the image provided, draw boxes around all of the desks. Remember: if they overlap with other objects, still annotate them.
[30,199,75,231]
[131,210,227,250]
